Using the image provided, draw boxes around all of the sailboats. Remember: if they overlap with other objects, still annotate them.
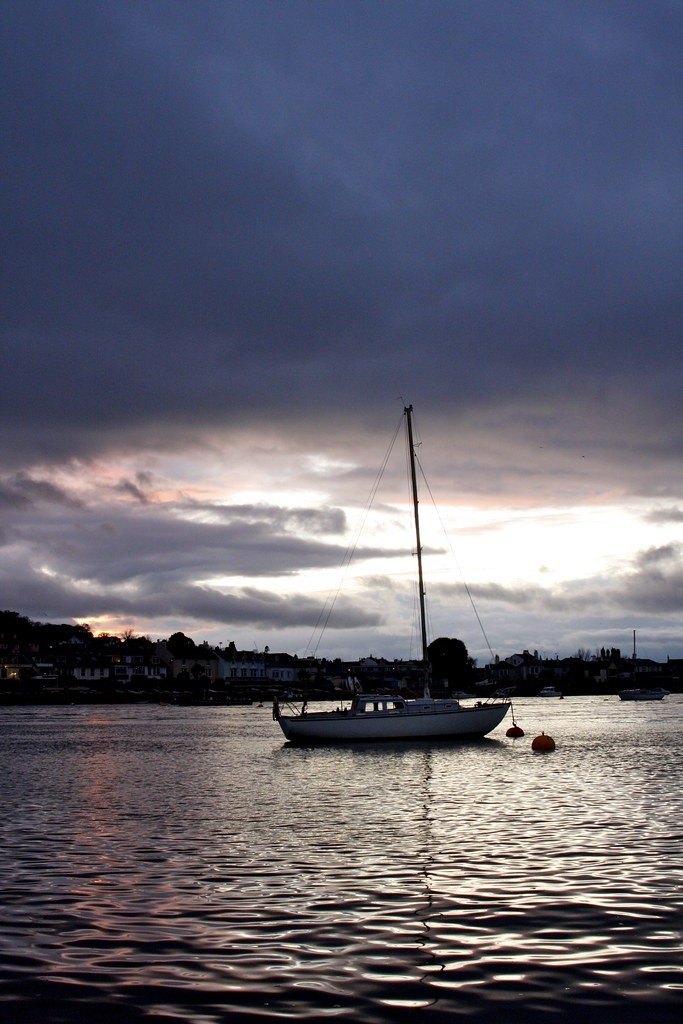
[272,403,511,740]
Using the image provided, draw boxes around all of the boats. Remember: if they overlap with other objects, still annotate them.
[617,689,665,700]
[539,687,561,697]
